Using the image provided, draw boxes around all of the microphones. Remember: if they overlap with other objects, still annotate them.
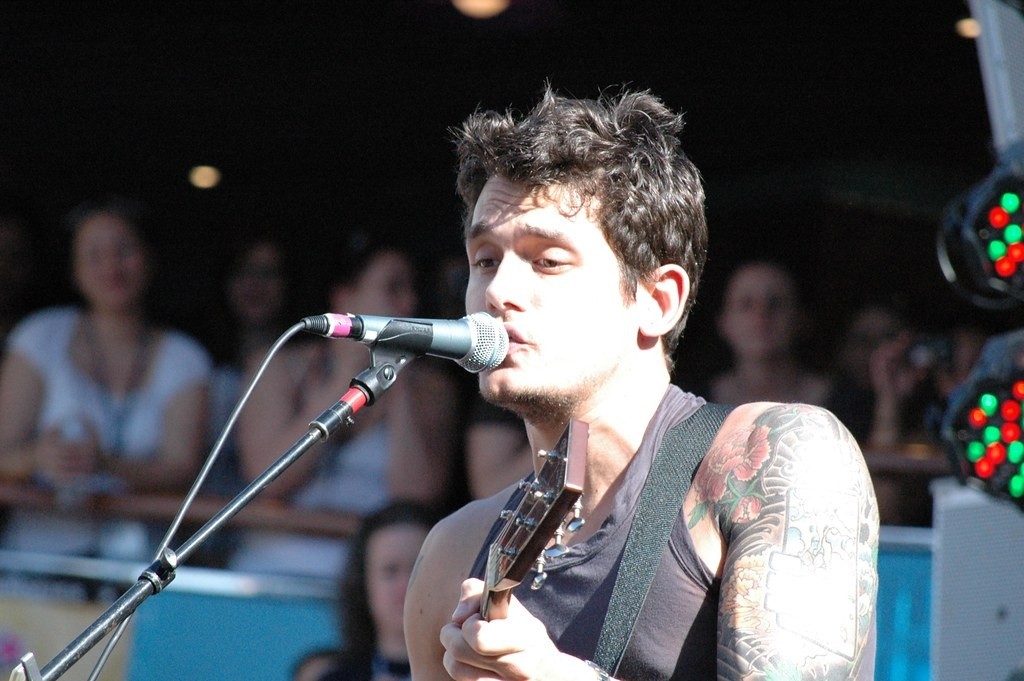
[301,312,510,373]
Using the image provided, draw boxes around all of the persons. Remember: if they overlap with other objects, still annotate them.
[402,87,886,681]
[0,198,981,681]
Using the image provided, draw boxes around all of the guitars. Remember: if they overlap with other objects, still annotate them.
[466,414,589,621]
